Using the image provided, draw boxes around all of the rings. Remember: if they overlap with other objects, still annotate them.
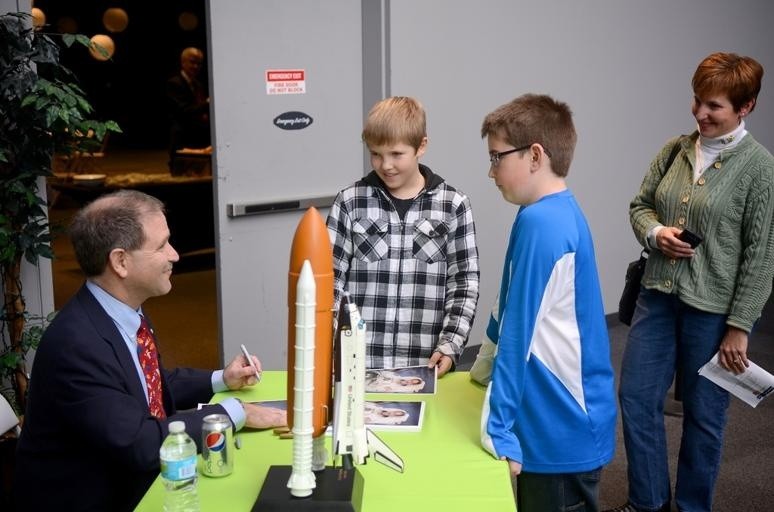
[734,358,740,364]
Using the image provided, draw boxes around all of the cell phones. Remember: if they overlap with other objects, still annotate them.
[678,228,704,250]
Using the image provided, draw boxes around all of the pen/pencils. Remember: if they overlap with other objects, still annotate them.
[241,344,261,381]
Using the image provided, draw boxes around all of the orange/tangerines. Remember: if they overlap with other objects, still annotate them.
[90,34,113,61]
[32,7,44,29]
[181,48,203,72]
[104,9,128,31]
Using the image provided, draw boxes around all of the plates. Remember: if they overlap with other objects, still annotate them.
[72,172,107,187]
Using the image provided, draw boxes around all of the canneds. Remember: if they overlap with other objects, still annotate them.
[201,415,233,478]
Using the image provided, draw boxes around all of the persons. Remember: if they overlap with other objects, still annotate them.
[325,96,479,379]
[363,402,409,425]
[365,372,426,393]
[470,93,619,512]
[14,189,288,512]
[164,47,209,176]
[617,51,774,511]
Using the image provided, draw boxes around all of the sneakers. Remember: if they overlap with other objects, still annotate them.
[602,502,671,512]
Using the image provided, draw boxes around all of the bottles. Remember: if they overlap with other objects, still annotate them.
[159,421,200,512]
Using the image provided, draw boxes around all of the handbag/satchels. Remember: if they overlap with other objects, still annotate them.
[616,253,649,329]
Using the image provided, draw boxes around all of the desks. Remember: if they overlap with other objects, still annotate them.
[131,367,517,509]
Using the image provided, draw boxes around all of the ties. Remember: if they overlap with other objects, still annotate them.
[133,315,167,421]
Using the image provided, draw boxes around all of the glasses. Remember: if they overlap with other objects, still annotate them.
[489,141,554,167]
